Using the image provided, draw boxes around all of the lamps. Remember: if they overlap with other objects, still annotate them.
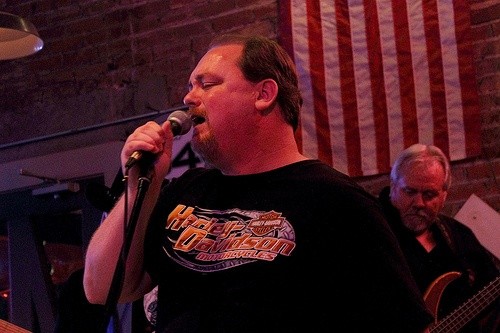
[0,11,44,61]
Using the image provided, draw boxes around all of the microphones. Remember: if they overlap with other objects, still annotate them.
[125,110,191,171]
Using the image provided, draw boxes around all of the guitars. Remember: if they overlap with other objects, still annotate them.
[421,271,500,333]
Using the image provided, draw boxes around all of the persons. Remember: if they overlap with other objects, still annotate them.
[82,32,436,333]
[374,143,500,333]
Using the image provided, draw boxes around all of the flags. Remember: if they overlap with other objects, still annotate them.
[276,0,482,178]
